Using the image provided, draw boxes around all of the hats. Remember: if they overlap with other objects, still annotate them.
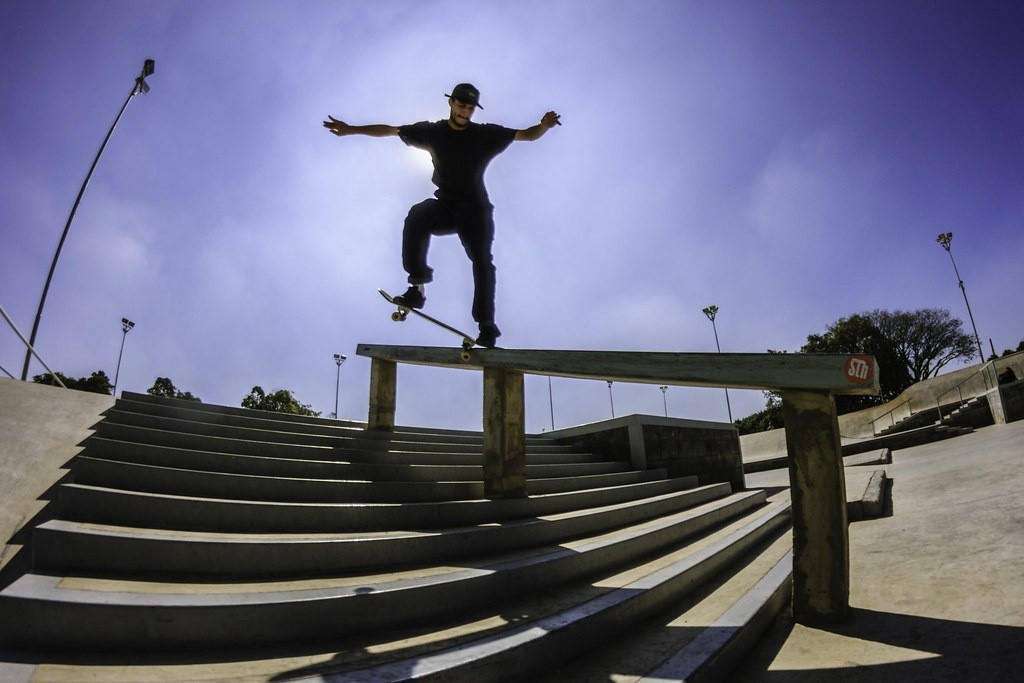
[445,83,483,110]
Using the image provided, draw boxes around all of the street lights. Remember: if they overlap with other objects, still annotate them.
[937,231,985,363]
[702,305,733,424]
[606,380,615,419]
[113,317,136,397]
[333,353,348,420]
[659,385,669,418]
[21,56,158,382]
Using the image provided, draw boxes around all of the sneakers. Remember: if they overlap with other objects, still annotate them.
[476,327,496,348]
[393,286,426,309]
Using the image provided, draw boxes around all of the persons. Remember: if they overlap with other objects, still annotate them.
[324,84,561,349]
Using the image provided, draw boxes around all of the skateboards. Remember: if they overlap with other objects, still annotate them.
[377,284,504,361]
